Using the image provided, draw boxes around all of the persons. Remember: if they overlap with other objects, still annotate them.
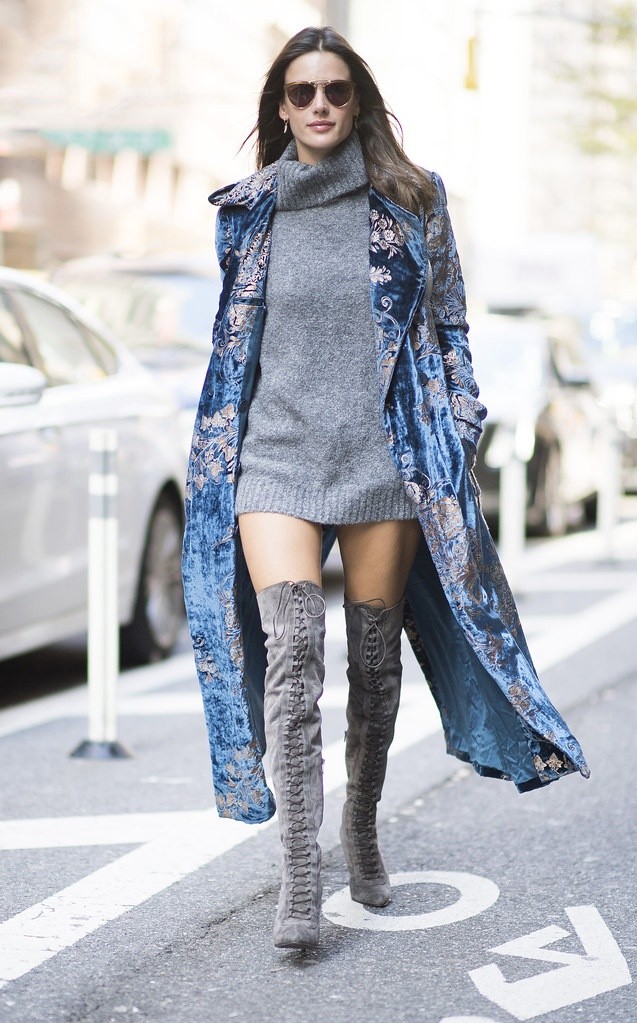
[177,26,589,956]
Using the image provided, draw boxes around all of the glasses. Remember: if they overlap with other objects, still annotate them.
[281,79,355,108]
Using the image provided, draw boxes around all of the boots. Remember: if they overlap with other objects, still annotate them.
[340,590,406,907]
[256,580,327,948]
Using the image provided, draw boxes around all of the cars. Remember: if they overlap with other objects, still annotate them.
[464,297,627,548]
[44,249,237,417]
[0,262,216,663]
